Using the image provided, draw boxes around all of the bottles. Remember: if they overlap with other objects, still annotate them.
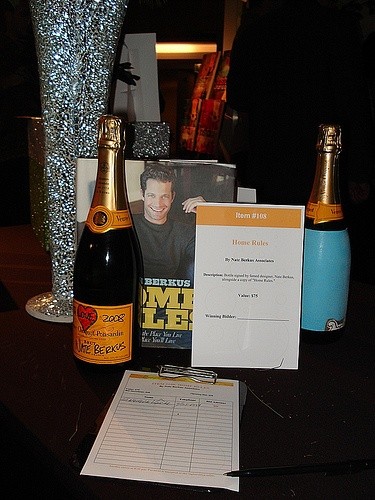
[300,125,352,337]
[71,116,143,375]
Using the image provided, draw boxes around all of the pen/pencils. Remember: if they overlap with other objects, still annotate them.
[223,460,375,477]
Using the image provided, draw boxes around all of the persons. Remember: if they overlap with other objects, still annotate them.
[131,162,207,277]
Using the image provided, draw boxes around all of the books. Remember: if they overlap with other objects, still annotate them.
[73,158,238,355]
